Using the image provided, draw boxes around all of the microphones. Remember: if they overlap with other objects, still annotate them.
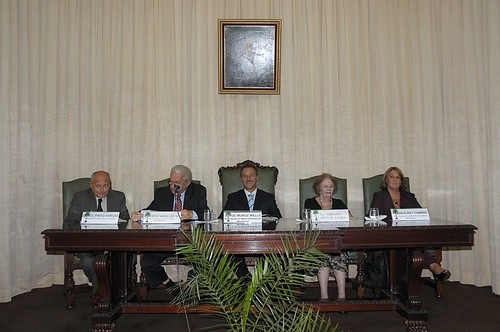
[395,202,398,204]
[176,185,184,223]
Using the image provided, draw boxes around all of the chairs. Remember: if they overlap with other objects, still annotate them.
[62,178,92,310]
[363,175,443,298]
[137,180,199,301]
[218,160,288,288]
[298,175,365,299]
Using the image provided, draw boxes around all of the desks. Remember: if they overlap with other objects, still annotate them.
[41,218,478,332]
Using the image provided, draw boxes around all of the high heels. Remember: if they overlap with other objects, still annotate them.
[434,269,450,282]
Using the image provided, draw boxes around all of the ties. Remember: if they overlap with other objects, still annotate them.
[247,194,254,210]
[97,199,103,211]
[177,194,183,210]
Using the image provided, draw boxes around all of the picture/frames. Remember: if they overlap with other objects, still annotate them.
[218,19,282,96]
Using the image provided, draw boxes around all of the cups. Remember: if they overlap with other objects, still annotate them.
[370,208,380,219]
[302,209,311,220]
[204,210,213,221]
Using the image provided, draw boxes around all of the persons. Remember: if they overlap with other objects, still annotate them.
[218,165,282,280]
[131,165,210,288]
[371,167,451,281]
[65,171,137,293]
[304,174,350,299]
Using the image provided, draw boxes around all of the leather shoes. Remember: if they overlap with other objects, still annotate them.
[91,295,101,306]
